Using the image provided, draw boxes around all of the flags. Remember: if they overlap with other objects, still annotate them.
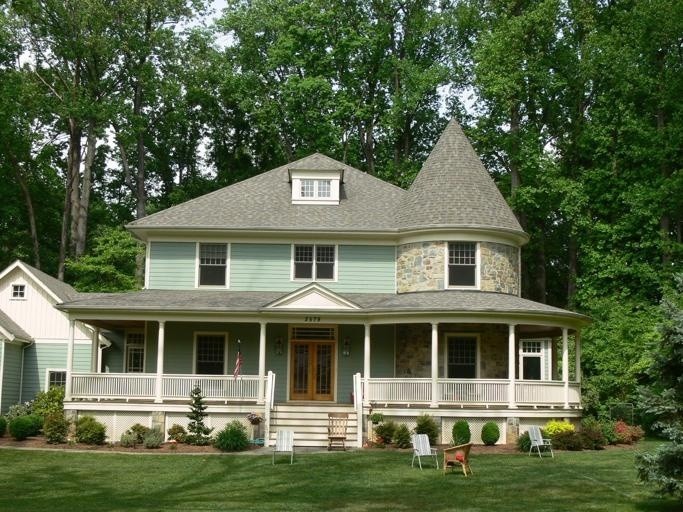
[233,351,243,381]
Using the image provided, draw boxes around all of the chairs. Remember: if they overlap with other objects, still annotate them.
[407,433,473,477]
[526,424,553,461]
[270,427,295,465]
[325,413,349,452]
